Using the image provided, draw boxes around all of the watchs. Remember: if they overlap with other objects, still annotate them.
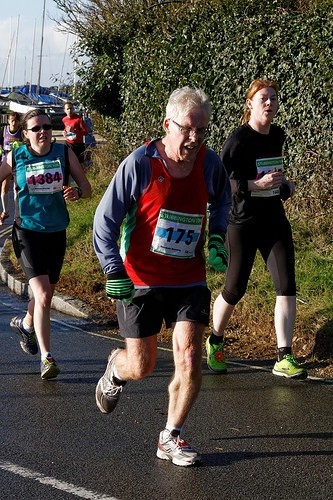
[75,187,82,200]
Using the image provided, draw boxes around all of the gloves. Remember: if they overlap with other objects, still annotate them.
[207,235,230,271]
[105,274,135,303]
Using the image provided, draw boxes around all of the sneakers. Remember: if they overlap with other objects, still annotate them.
[205,335,227,372]
[40,353,60,381]
[95,346,127,413]
[272,354,308,379]
[9,315,39,355]
[156,431,197,466]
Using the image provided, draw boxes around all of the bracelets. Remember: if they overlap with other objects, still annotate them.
[237,179,248,193]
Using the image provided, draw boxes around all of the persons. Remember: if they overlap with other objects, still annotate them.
[94,85,232,466]
[0,112,24,224]
[205,81,309,380]
[0,110,92,380]
[62,102,87,163]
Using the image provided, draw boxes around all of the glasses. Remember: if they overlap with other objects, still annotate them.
[168,119,209,135]
[25,124,52,132]
